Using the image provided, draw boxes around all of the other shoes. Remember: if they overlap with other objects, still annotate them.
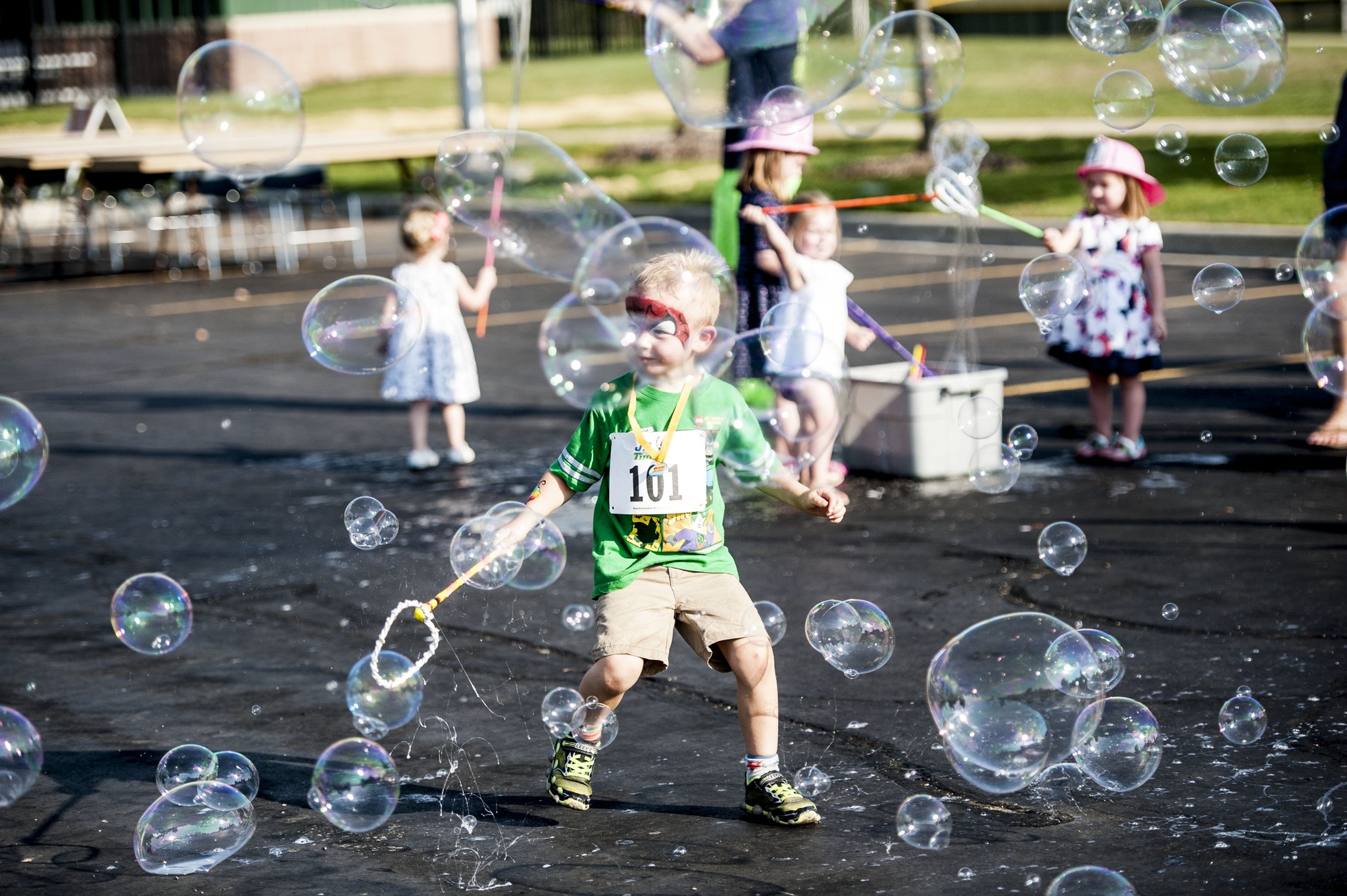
[407,448,439,468]
[1098,435,1148,460]
[1074,434,1110,457]
[447,441,476,464]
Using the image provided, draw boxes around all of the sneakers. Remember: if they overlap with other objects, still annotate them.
[742,771,822,825]
[545,730,601,810]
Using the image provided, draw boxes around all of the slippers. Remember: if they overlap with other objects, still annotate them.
[1307,425,1347,450]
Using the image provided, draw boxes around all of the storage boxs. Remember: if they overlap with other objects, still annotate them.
[840,360,1007,480]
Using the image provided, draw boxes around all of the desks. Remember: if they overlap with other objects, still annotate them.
[0,133,501,288]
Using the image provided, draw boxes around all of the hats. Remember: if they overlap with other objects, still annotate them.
[1075,136,1164,204]
[728,97,819,154]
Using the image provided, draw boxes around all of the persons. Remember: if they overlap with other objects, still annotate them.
[374,199,496,472]
[1309,73,1347,448]
[1041,137,1167,463]
[492,248,846,826]
[611,0,874,493]
